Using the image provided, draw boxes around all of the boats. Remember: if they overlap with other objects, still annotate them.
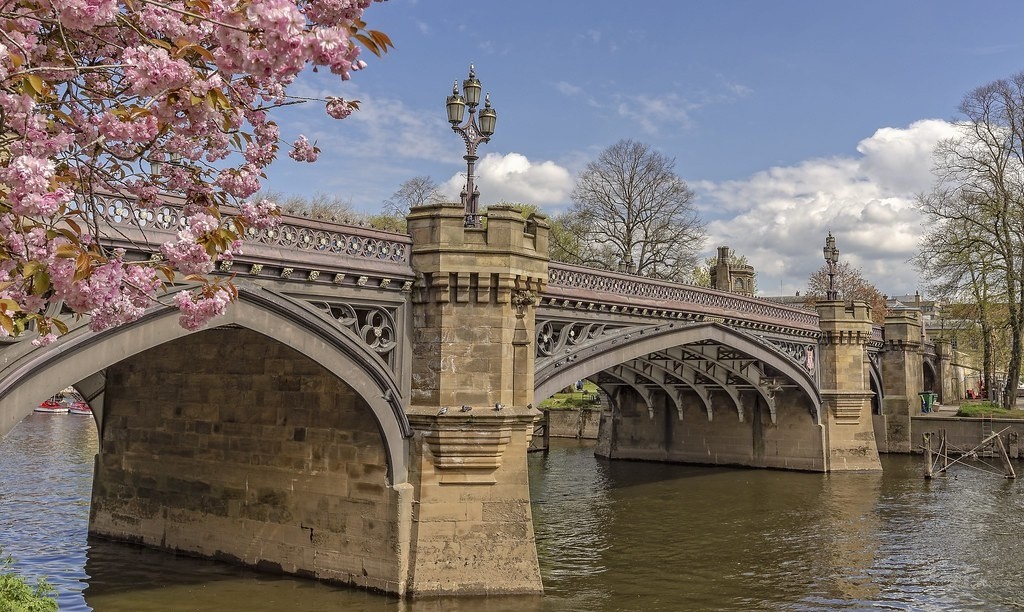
[34,395,70,413]
[66,399,94,415]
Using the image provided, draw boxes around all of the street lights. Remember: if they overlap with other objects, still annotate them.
[446,64,498,228]
[824,230,840,301]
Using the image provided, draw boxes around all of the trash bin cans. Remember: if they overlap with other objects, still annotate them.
[918,391,933,412]
[932,394,939,403]
